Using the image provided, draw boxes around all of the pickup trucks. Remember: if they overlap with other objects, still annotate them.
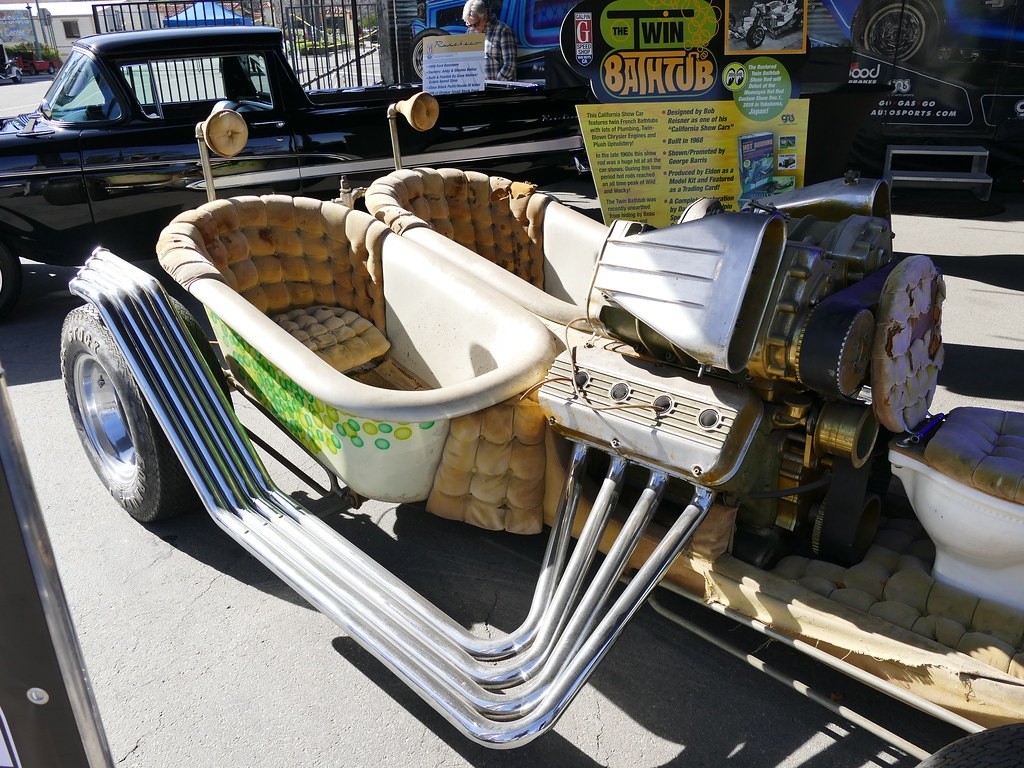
[0,27,568,324]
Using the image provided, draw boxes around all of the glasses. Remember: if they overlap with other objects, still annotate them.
[465,16,482,27]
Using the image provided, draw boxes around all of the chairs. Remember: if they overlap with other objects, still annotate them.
[867,255,1024,611]
[365,167,553,291]
[153,195,391,374]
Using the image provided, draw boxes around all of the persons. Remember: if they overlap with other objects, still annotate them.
[461,0,517,83]
